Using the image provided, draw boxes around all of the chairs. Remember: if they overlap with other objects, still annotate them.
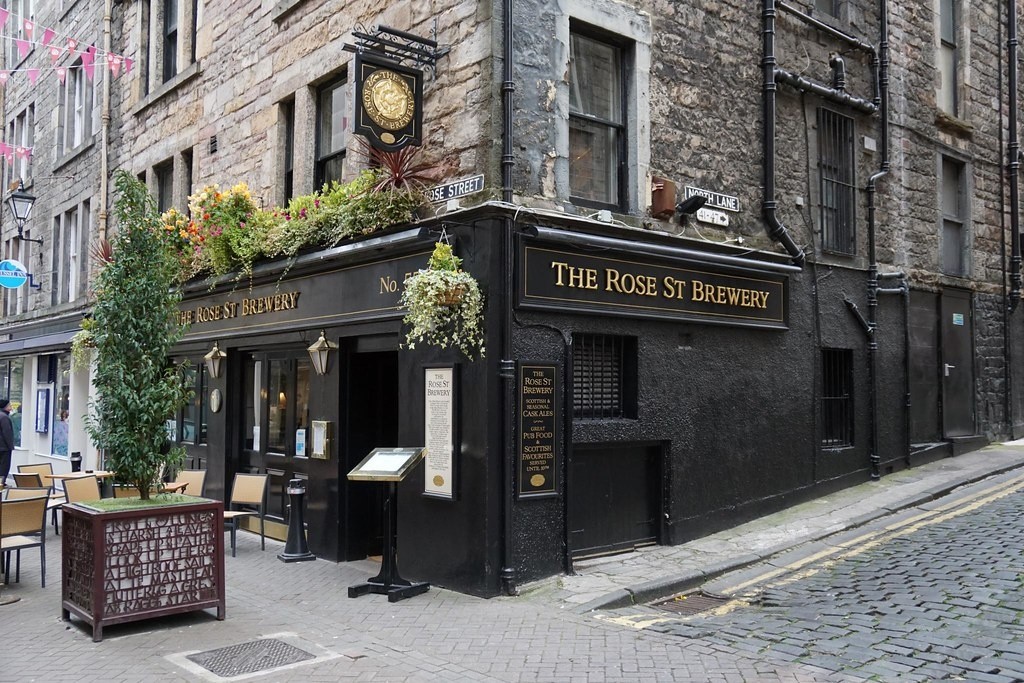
[62,475,101,503]
[174,467,208,497]
[17,463,65,525]
[13,473,67,535]
[223,473,269,558]
[0,495,49,588]
[5,486,52,583]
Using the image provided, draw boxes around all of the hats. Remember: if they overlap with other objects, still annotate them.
[0,399,9,408]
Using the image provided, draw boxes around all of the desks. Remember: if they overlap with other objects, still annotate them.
[44,470,116,480]
[156,482,189,494]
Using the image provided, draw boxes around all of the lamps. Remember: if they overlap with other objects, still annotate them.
[675,196,708,227]
[306,329,337,376]
[4,174,44,245]
[202,341,226,379]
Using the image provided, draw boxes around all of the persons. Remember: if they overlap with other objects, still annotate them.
[0,399,15,489]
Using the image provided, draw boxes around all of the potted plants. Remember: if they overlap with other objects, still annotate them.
[400,269,487,360]
[58,169,225,641]
